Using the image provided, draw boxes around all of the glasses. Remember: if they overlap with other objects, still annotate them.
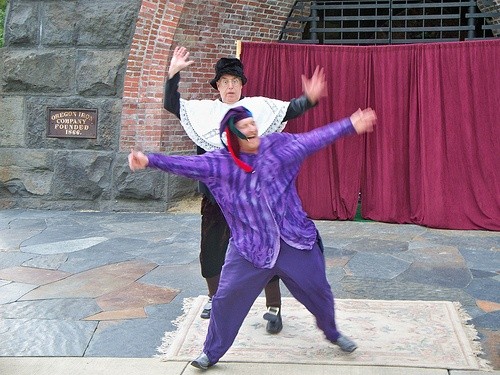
[216,78,241,86]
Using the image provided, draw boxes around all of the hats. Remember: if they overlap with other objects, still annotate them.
[220,105,256,172]
[208,57,248,89]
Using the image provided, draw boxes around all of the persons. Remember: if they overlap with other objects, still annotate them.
[127,106,377,370]
[164,46,327,333]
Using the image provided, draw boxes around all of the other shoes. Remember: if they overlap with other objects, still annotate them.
[200,298,215,319]
[330,334,358,352]
[263,311,283,333]
[191,352,213,371]
